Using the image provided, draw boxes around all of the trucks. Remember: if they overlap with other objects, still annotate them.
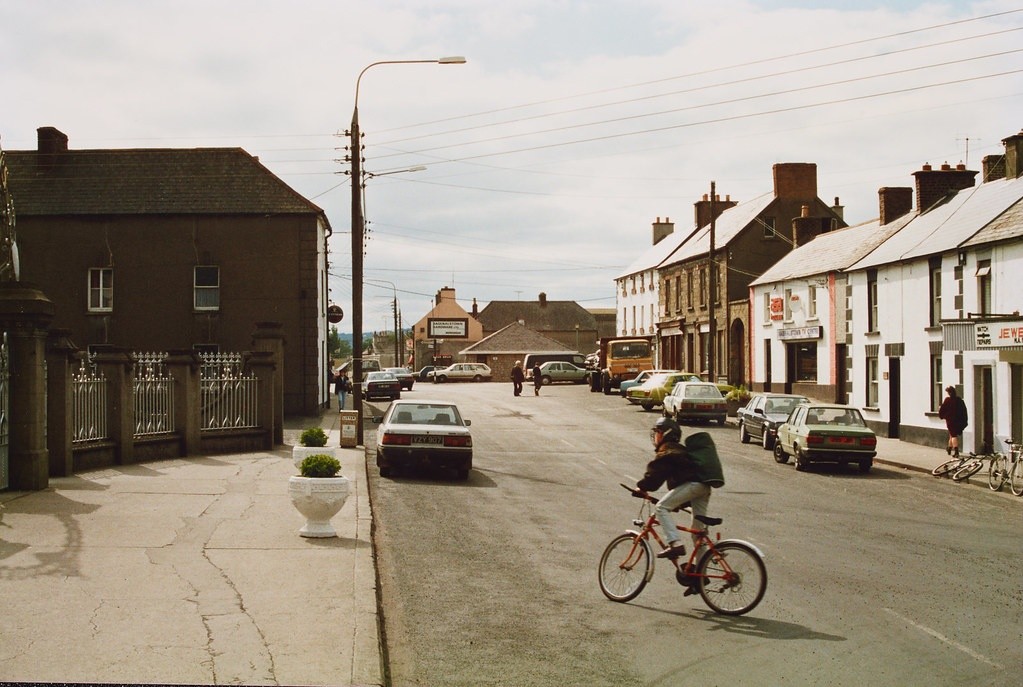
[585,335,656,395]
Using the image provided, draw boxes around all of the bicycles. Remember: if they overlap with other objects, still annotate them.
[597,481,767,616]
[987,439,1023,496]
[932,450,998,482]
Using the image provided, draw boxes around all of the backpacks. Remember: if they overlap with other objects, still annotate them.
[684,431,725,488]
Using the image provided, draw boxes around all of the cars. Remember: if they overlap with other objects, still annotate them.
[361,372,401,400]
[426,363,493,382]
[773,402,876,472]
[526,361,597,385]
[663,383,727,426]
[372,401,474,481]
[736,395,810,450]
[382,369,414,391]
[620,370,738,410]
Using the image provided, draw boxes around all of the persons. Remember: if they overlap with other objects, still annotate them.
[533,362,541,395]
[632,416,710,597]
[511,360,524,396]
[334,369,348,412]
[938,386,960,457]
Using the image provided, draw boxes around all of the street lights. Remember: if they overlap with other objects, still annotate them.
[351,55,467,443]
[365,278,404,367]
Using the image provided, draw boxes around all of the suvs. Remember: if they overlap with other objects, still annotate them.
[419,366,449,382]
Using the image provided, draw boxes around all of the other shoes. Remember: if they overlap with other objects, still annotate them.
[953,452,959,457]
[656,544,687,558]
[946,446,951,454]
[684,576,711,596]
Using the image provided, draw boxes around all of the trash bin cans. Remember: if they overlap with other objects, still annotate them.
[340,409,358,448]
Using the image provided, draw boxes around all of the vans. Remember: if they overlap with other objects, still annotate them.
[346,357,381,392]
[523,352,587,381]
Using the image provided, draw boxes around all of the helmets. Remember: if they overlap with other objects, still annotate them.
[649,416,682,452]
